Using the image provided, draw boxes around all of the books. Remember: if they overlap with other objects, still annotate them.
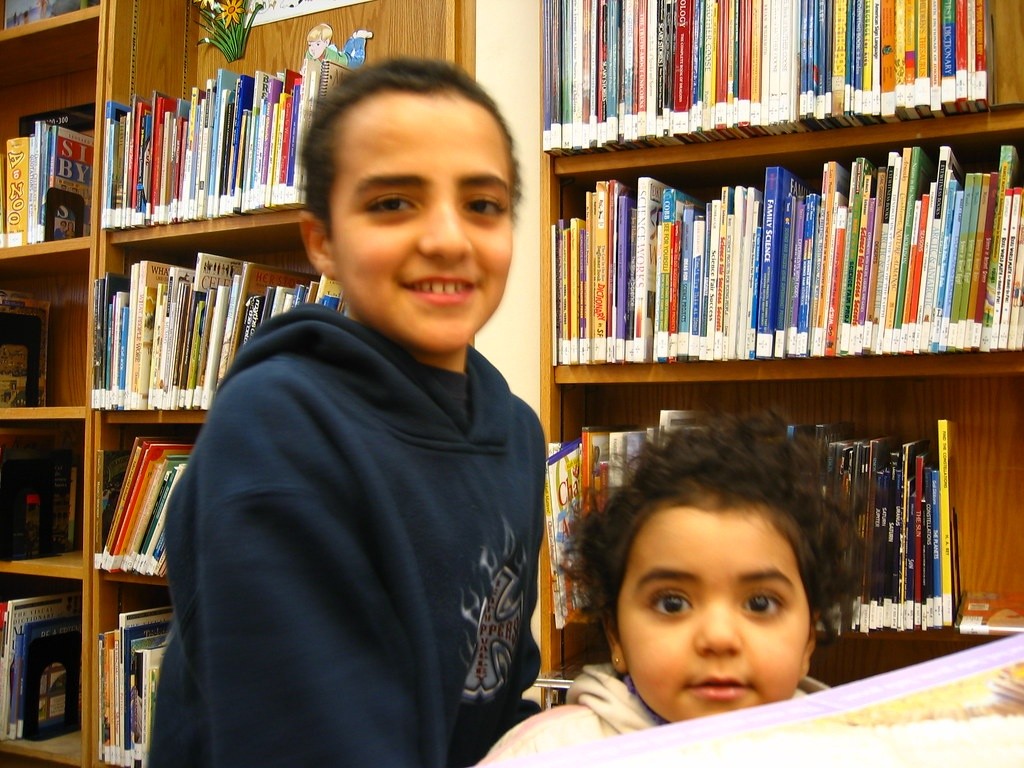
[0,101,98,249]
[950,589,1023,635]
[0,425,78,563]
[92,249,354,411]
[95,602,177,768]
[0,584,81,743]
[548,413,962,633]
[91,429,199,578]
[549,135,1024,363]
[542,0,997,153]
[101,55,359,232]
[0,287,51,410]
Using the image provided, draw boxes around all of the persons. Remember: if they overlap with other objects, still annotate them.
[136,53,548,768]
[467,399,872,767]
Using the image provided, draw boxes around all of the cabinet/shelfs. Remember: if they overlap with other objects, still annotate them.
[0,0,110,768]
[90,0,477,768]
[539,1,1024,712]
